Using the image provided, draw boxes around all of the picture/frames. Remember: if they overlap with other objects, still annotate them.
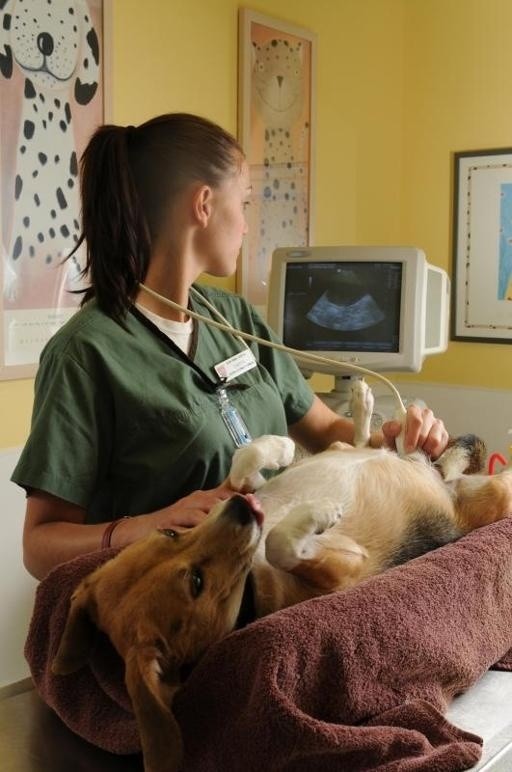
[230,2,322,330]
[0,1,118,385]
[449,145,510,347]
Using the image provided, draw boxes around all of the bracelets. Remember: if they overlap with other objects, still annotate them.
[103,514,130,549]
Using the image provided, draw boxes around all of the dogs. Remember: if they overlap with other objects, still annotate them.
[50,372,512,771]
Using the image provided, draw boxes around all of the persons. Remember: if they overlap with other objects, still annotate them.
[10,112,451,585]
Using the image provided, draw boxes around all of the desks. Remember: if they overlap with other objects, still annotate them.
[433,663,512,772]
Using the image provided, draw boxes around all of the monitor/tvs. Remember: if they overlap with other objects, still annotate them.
[265,245,451,376]
